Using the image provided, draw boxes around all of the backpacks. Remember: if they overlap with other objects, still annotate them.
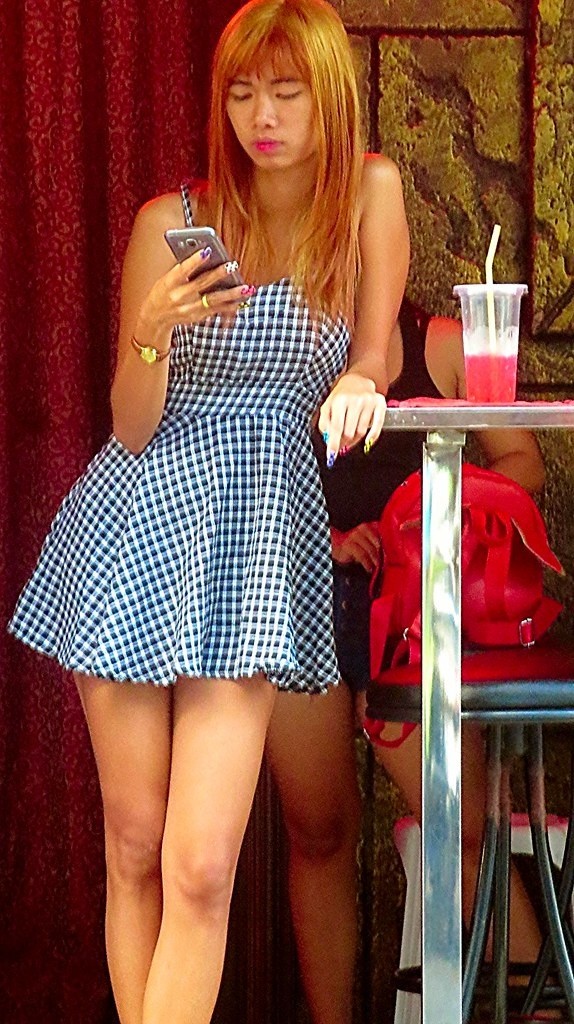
[362,463,567,748]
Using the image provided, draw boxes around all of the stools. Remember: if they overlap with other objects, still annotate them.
[394,813,574,1024]
[366,646,574,1024]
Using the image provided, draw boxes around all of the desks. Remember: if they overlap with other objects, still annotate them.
[371,409,574,1024]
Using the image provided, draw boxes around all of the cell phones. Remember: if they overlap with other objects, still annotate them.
[165,226,243,295]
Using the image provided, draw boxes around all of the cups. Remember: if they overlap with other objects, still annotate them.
[453,284,528,403]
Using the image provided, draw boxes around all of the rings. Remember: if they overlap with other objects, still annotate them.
[202,294,209,308]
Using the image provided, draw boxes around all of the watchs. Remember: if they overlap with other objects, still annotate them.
[130,336,171,364]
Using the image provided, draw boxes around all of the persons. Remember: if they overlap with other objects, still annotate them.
[5,0,410,1024]
[265,295,545,1024]
